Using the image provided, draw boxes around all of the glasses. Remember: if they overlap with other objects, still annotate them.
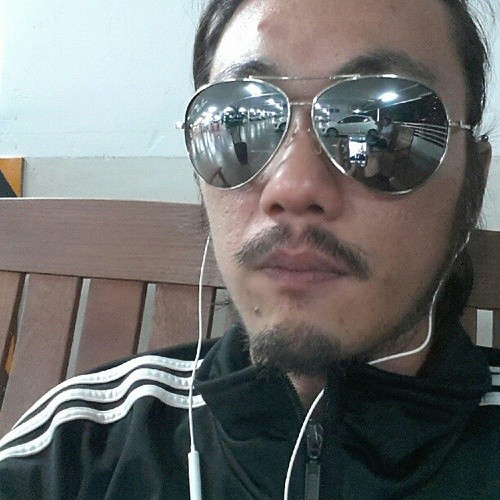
[174,73,476,195]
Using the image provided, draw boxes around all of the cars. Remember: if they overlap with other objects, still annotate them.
[218,109,273,123]
[317,113,379,137]
[269,115,289,132]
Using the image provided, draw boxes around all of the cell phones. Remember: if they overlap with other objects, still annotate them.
[348,133,368,168]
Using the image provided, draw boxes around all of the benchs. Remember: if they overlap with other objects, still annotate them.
[0,200,500,437]
[366,122,416,163]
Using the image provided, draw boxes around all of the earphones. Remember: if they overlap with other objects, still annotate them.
[463,193,475,232]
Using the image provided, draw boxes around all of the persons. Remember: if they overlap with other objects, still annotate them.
[365,117,394,153]
[0,0,500,500]
[264,113,272,124]
